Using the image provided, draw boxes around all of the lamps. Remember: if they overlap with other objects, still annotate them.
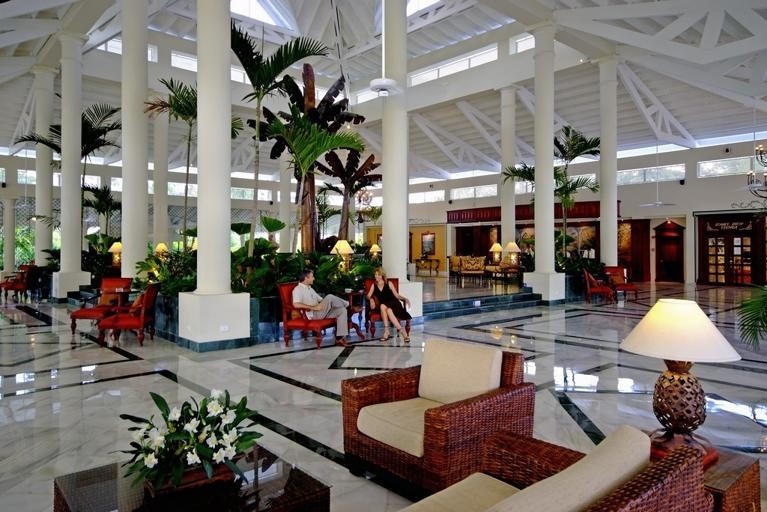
[503,242,521,266]
[154,243,169,263]
[370,243,382,263]
[490,243,503,261]
[107,242,123,267]
[329,240,354,276]
[618,299,742,462]
[747,145,767,199]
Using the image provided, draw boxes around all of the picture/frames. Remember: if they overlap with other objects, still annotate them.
[420,233,436,255]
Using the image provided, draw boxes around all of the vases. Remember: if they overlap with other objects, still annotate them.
[142,452,247,503]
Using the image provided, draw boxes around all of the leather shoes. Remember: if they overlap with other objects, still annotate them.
[351,307,363,312]
[335,338,352,348]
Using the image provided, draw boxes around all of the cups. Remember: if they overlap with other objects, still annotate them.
[345,289,353,293]
[116,288,123,292]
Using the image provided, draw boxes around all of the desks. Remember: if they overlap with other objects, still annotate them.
[99,288,141,341]
[336,291,365,346]
[504,267,524,288]
[487,272,504,285]
[415,258,439,276]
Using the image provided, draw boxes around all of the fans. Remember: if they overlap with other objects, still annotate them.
[638,139,677,209]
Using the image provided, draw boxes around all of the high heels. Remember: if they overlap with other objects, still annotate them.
[380,326,390,341]
[397,328,409,342]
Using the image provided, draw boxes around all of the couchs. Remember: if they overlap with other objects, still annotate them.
[395,424,714,511]
[339,336,536,493]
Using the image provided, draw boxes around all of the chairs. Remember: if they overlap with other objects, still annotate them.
[582,268,613,305]
[277,282,336,350]
[363,279,411,338]
[98,284,159,348]
[604,266,640,301]
[446,256,462,286]
[71,278,132,342]
[1,265,32,300]
[462,256,485,289]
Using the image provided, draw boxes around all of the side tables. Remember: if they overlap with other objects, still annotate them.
[636,427,760,512]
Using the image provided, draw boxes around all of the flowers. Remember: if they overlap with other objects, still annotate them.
[107,390,264,485]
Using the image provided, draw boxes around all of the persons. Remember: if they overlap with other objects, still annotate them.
[291,269,362,347]
[366,268,413,344]
